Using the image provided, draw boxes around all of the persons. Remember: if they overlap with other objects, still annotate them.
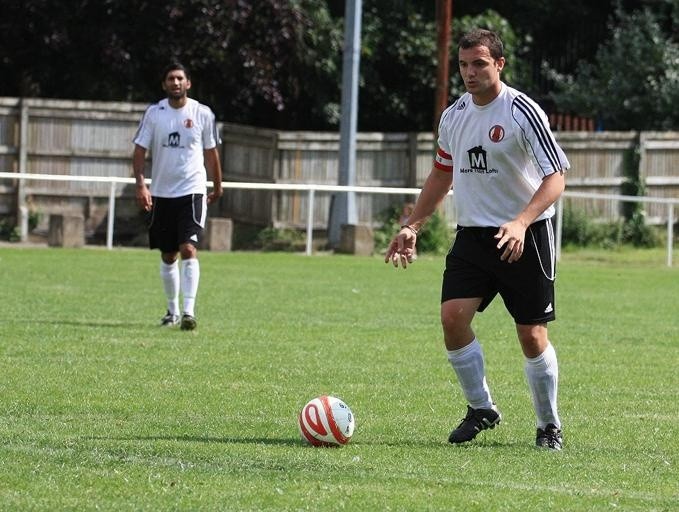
[386,27,573,449]
[133,62,222,331]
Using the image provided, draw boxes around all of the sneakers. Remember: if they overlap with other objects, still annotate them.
[536,422,563,451]
[179,315,198,331]
[160,311,179,328]
[448,402,503,444]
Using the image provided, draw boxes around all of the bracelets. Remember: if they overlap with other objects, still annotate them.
[402,225,419,235]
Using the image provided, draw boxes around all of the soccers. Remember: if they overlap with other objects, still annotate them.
[299,396,355,447]
